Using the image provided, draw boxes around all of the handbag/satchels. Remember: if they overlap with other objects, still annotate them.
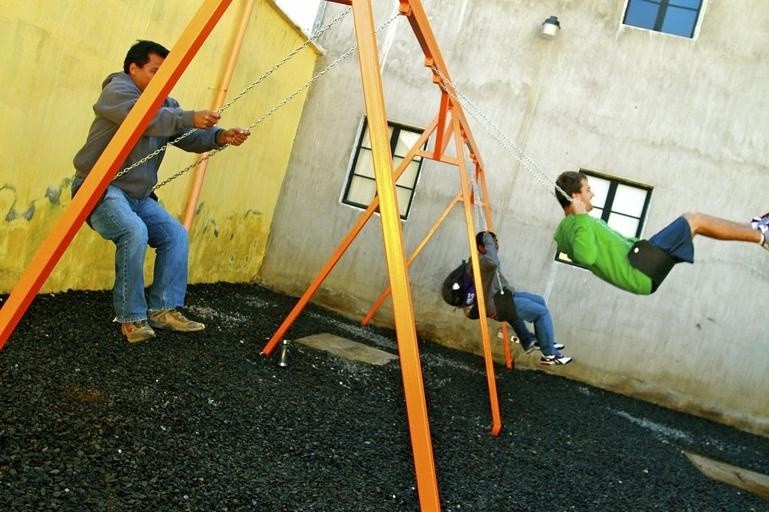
[442,263,475,306]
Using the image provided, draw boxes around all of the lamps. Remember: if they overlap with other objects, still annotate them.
[542,16,562,38]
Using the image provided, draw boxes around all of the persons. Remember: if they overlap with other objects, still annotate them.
[70,41,251,344]
[442,230,572,366]
[553,170,768,295]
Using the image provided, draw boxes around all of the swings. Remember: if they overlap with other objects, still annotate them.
[464,136,518,322]
[431,63,674,283]
[73,5,403,230]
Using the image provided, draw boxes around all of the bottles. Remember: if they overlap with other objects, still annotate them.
[452,280,461,306]
[277,339,290,368]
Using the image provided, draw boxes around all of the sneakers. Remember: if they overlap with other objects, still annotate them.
[147,308,205,333]
[121,321,155,343]
[751,213,769,251]
[526,340,566,354]
[540,354,571,366]
